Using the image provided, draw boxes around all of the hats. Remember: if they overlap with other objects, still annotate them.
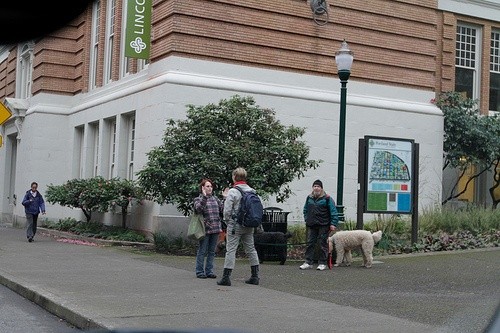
[313,180,323,188]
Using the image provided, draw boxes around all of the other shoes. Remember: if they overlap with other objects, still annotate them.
[29,238,32,242]
[197,275,207,278]
[208,274,217,278]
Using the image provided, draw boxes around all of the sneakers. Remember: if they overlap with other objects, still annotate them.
[299,262,313,269]
[317,264,329,270]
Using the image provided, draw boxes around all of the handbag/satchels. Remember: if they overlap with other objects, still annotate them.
[187,213,206,241]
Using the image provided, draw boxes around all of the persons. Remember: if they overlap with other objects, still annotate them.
[216,166,260,286]
[299,180,339,270]
[22,182,45,242]
[193,179,229,279]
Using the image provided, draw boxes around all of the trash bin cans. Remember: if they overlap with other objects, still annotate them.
[253,207,292,265]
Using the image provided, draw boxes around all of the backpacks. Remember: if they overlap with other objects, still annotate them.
[235,186,263,228]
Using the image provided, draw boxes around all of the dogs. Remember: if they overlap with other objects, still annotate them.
[327,230,382,269]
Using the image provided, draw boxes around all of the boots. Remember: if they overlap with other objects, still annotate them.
[217,268,233,286]
[246,265,259,285]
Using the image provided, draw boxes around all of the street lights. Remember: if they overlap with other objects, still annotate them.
[334,37,354,223]
